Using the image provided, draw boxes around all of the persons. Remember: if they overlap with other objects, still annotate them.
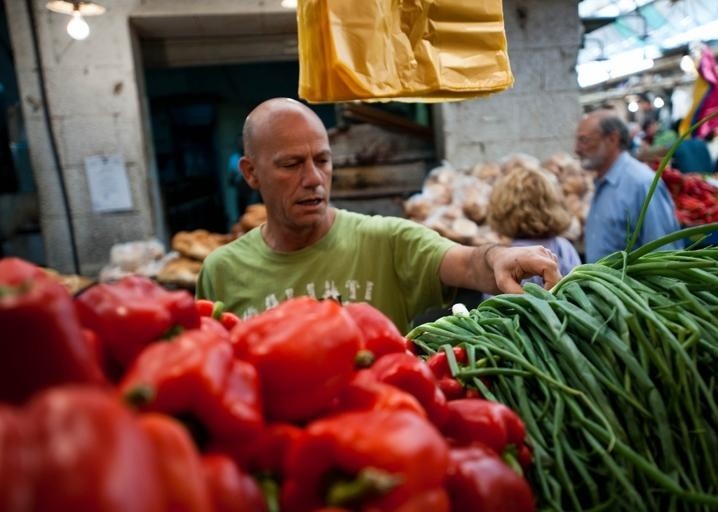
[194,97,562,339]
[573,106,685,265]
[482,165,582,305]
[624,112,718,176]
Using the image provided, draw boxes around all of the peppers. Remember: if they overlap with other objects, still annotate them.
[1,254,535,511]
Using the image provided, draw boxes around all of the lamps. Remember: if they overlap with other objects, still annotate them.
[46,0,105,41]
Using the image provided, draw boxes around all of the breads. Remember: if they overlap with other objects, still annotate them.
[98,202,270,284]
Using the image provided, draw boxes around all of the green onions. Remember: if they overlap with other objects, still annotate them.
[404,113,718,512]
